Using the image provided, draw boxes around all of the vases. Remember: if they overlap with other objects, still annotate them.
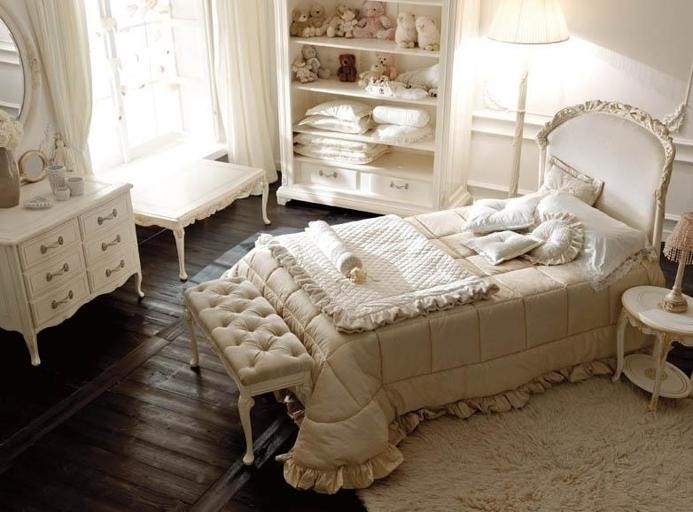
[0,147,20,208]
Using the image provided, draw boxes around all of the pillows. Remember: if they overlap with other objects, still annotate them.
[521,212,585,264]
[544,156,604,206]
[463,193,542,232]
[528,191,656,290]
[304,220,367,283]
[460,230,544,266]
[292,99,433,165]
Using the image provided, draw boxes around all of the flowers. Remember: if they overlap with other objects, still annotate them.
[0,110,24,148]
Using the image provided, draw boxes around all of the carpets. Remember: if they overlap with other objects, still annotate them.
[355,373,692,512]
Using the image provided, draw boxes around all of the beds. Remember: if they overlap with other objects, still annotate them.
[220,100,676,494]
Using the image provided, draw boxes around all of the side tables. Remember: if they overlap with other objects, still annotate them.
[611,284,693,414]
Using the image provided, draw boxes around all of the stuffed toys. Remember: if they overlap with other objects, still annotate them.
[287,0,441,100]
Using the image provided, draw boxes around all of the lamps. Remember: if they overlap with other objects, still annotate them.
[662,213,693,313]
[485,2,568,198]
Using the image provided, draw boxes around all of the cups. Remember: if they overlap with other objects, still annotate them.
[55,187,69,200]
[66,177,84,196]
[45,166,66,195]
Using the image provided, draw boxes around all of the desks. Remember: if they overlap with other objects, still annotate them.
[0,172,145,366]
[184,276,315,465]
[132,157,272,279]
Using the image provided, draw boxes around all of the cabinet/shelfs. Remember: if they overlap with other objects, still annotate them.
[273,0,474,217]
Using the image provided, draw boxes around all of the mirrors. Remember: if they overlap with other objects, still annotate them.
[19,150,48,183]
[0,0,42,144]
